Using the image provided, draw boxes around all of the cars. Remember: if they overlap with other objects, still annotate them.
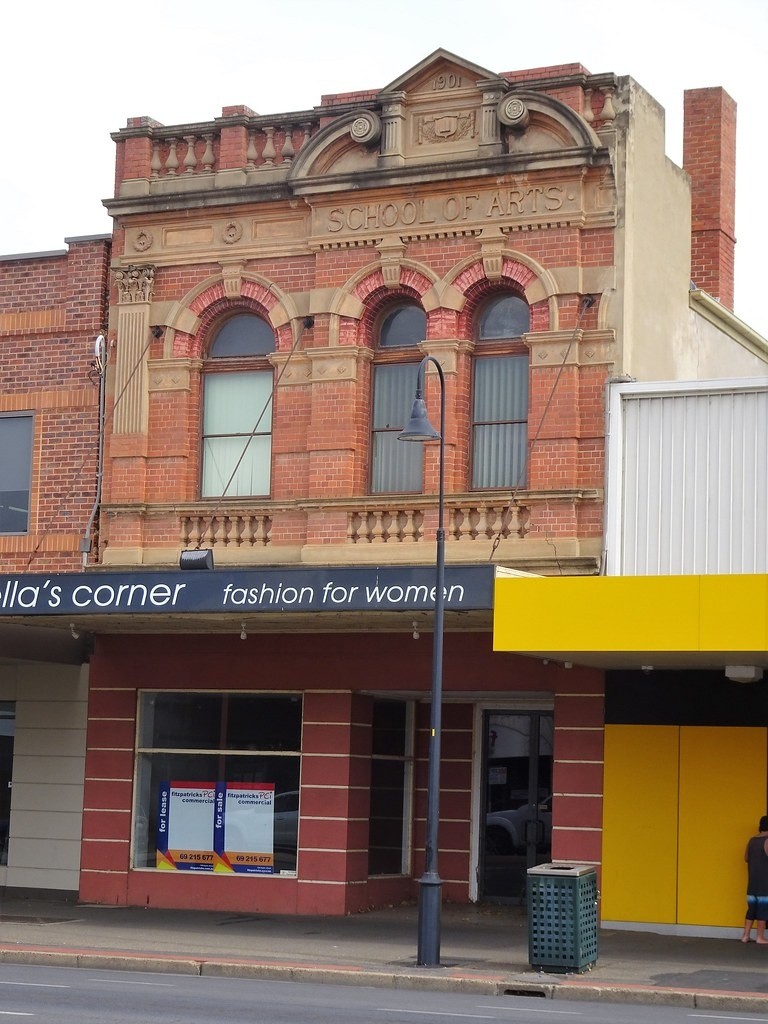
[226,791,299,853]
[482,795,551,854]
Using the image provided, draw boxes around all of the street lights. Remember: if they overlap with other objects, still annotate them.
[395,356,442,963]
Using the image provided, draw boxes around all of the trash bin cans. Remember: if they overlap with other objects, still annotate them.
[525,862,599,976]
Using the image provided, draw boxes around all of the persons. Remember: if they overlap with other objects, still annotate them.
[741,815,768,943]
